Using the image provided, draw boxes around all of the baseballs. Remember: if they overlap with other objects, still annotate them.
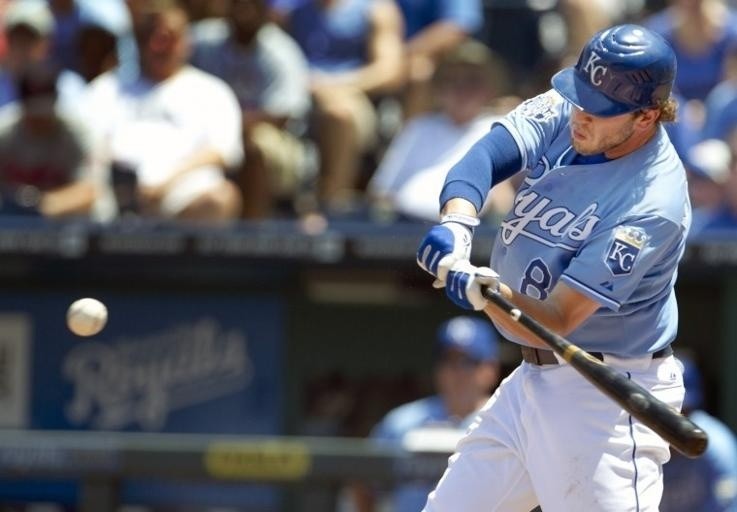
[67,298,109,336]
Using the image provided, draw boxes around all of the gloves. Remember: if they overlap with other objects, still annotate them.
[446,260,501,312]
[417,210,480,288]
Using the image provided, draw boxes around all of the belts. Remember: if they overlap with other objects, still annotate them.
[521,336,673,364]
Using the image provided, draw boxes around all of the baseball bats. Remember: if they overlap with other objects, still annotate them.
[482,284,708,458]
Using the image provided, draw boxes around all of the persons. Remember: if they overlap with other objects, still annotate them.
[660,345,737,511]
[0,0,737,237]
[416,23,688,512]
[329,316,504,511]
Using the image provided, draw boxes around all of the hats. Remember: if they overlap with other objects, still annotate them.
[6,2,54,38]
[441,317,501,361]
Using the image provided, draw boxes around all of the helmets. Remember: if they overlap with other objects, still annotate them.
[551,24,677,118]
